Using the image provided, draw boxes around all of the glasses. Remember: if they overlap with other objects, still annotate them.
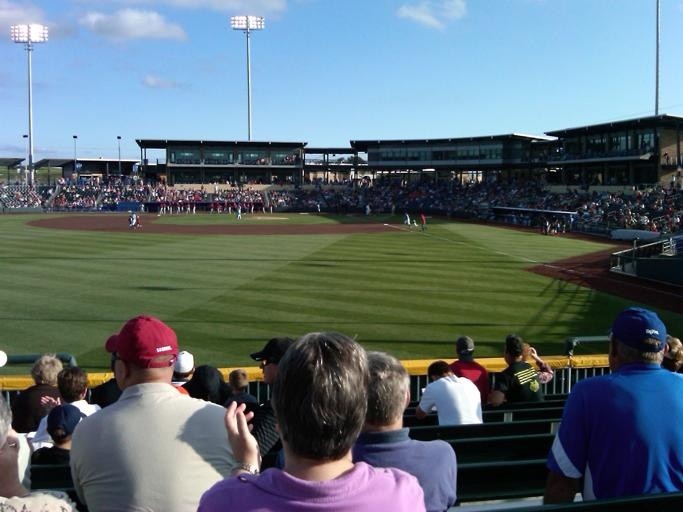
[262,359,271,365]
[111,352,121,361]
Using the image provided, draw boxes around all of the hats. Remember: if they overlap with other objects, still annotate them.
[0,350,7,367]
[456,336,474,355]
[106,316,178,368]
[174,350,195,373]
[608,305,667,353]
[250,337,295,365]
[46,405,88,440]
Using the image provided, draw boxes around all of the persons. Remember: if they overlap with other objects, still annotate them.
[43,176,102,213]
[0,394,79,512]
[0,181,43,208]
[665,151,670,164]
[274,176,296,185]
[402,212,411,228]
[167,175,225,215]
[543,306,683,503]
[660,333,683,373]
[449,335,490,404]
[274,188,325,208]
[197,329,426,512]
[521,342,554,384]
[330,177,351,186]
[364,176,490,214]
[281,153,299,165]
[90,378,122,409]
[490,173,683,236]
[351,351,457,512]
[224,369,257,408]
[190,364,232,407]
[128,212,143,232]
[304,176,328,186]
[522,147,551,161]
[226,175,274,220]
[102,173,167,216]
[70,314,262,512]
[642,140,646,154]
[418,213,427,231]
[415,361,484,425]
[249,337,295,385]
[255,157,265,165]
[488,334,545,404]
[325,188,364,208]
[31,365,102,445]
[11,351,64,433]
[173,350,196,382]
[30,404,87,512]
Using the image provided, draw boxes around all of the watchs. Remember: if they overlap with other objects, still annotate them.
[231,462,260,476]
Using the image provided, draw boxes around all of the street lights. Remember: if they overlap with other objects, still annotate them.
[73,135,77,173]
[116,135,122,173]
[22,135,29,186]
